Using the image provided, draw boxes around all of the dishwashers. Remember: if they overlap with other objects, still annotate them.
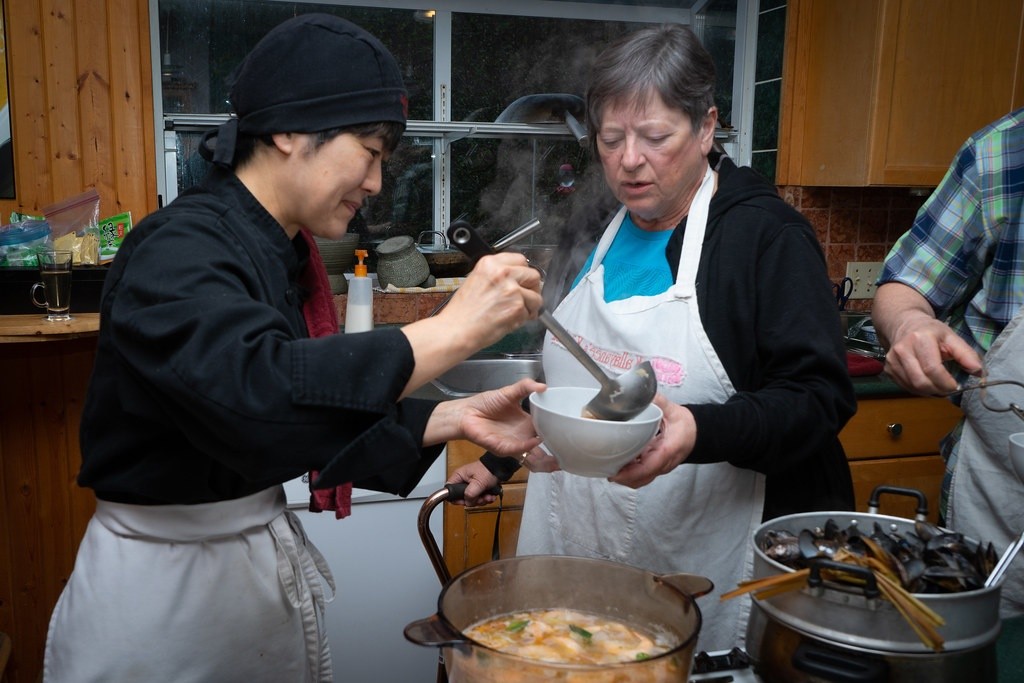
[277,444,446,682]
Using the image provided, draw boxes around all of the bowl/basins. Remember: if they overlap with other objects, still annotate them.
[863,326,884,351]
[1009,433,1023,484]
[374,235,430,290]
[531,384,663,478]
[312,233,361,295]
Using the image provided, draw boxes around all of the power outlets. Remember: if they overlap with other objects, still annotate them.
[845,262,882,299]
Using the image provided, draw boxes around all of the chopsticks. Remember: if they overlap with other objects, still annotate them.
[985,530,1023,589]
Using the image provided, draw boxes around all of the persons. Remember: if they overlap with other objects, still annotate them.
[446,22,859,661]
[39,13,558,682]
[870,109,1022,619]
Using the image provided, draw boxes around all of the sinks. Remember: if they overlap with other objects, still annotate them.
[424,358,571,403]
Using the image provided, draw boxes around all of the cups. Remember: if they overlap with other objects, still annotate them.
[30,249,74,322]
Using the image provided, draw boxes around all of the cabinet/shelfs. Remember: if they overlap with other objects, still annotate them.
[840,396,967,524]
[776,0,1024,188]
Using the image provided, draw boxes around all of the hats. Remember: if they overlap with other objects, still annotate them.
[197,12,408,164]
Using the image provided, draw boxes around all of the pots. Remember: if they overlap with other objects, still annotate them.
[403,556,715,683]
[413,230,470,277]
[744,484,1001,683]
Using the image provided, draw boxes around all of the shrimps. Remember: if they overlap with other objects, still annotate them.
[466,608,677,681]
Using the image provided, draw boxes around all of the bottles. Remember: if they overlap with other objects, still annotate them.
[342,249,377,333]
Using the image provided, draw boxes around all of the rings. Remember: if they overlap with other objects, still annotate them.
[519,451,531,466]
